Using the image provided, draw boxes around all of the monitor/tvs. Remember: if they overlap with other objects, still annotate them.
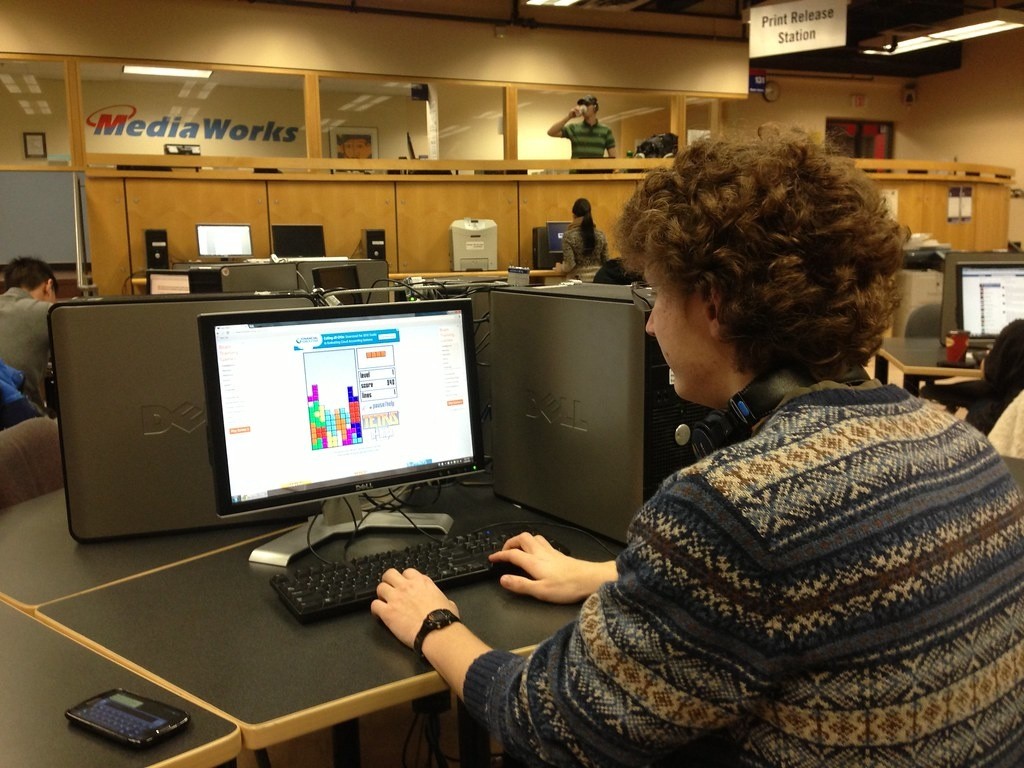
[146,269,190,294]
[312,265,363,305]
[196,297,487,566]
[271,225,327,257]
[546,220,572,253]
[195,223,255,258]
[955,261,1023,338]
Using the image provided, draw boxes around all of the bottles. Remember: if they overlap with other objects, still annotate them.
[626,150,634,159]
[409,294,416,301]
[43,362,58,417]
[507,266,530,284]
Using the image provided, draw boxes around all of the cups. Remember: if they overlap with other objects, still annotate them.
[945,331,971,362]
[575,105,588,117]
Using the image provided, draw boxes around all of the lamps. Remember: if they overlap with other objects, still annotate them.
[858,0,1024,56]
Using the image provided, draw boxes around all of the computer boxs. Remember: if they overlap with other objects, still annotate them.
[532,227,563,269]
[49,292,322,542]
[940,251,1024,347]
[188,258,390,304]
[490,284,712,548]
[361,228,386,261]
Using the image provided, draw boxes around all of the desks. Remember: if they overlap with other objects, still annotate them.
[1,482,628,768]
[84,152,1016,295]
[875,335,982,398]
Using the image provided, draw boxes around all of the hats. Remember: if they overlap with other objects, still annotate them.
[577,95,598,105]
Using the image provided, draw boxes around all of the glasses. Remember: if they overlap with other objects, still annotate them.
[631,281,683,312]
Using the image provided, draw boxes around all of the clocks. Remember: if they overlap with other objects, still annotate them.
[764,80,782,101]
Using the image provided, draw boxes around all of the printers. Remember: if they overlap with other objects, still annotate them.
[449,219,496,272]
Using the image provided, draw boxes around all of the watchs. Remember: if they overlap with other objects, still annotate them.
[414,609,462,658]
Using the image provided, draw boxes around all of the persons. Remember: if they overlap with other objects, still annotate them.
[556,199,610,282]
[370,120,1024,768]
[548,95,617,174]
[0,256,58,414]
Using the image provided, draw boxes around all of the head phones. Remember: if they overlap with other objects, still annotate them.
[689,363,872,460]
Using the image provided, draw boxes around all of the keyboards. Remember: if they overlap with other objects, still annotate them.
[269,523,570,624]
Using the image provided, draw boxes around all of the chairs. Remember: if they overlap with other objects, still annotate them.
[905,302,957,385]
[0,416,64,511]
[987,388,1024,497]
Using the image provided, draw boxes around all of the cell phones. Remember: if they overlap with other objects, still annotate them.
[64,688,190,748]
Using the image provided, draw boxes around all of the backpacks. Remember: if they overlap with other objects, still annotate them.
[636,132,677,158]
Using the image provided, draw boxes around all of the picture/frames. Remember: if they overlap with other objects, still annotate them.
[330,126,378,176]
[23,132,47,159]
